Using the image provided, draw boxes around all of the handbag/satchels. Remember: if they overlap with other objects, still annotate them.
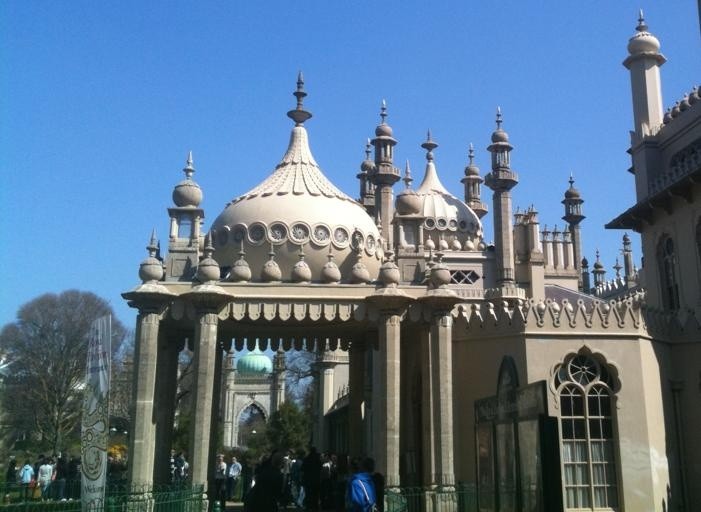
[368,503,379,512]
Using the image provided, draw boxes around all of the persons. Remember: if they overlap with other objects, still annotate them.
[170,447,189,481]
[226,456,243,500]
[215,453,227,499]
[3,444,128,504]
[243,445,384,512]
